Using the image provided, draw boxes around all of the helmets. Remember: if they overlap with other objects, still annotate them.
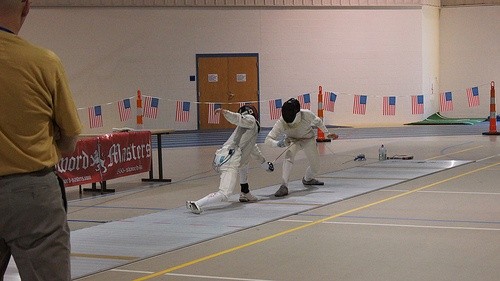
[237,104,258,120]
[281,98,300,124]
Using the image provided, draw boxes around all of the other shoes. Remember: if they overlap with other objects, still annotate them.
[239,191,258,202]
[302,176,324,185]
[185,200,202,214]
[274,185,289,197]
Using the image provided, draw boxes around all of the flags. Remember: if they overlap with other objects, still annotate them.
[411,95,425,114]
[239,102,252,108]
[297,93,311,110]
[269,98,283,120]
[439,92,453,114]
[175,100,190,123]
[143,96,159,120]
[118,98,132,121]
[88,104,103,128]
[208,103,221,124]
[353,94,367,115]
[383,96,396,116]
[322,92,337,112]
[467,86,481,108]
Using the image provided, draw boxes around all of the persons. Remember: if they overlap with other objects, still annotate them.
[185,105,275,214]
[0,0,81,281]
[264,98,339,197]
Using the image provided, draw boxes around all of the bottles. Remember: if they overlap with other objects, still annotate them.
[378,144,386,160]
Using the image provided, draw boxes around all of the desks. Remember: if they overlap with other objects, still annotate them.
[57,129,172,193]
